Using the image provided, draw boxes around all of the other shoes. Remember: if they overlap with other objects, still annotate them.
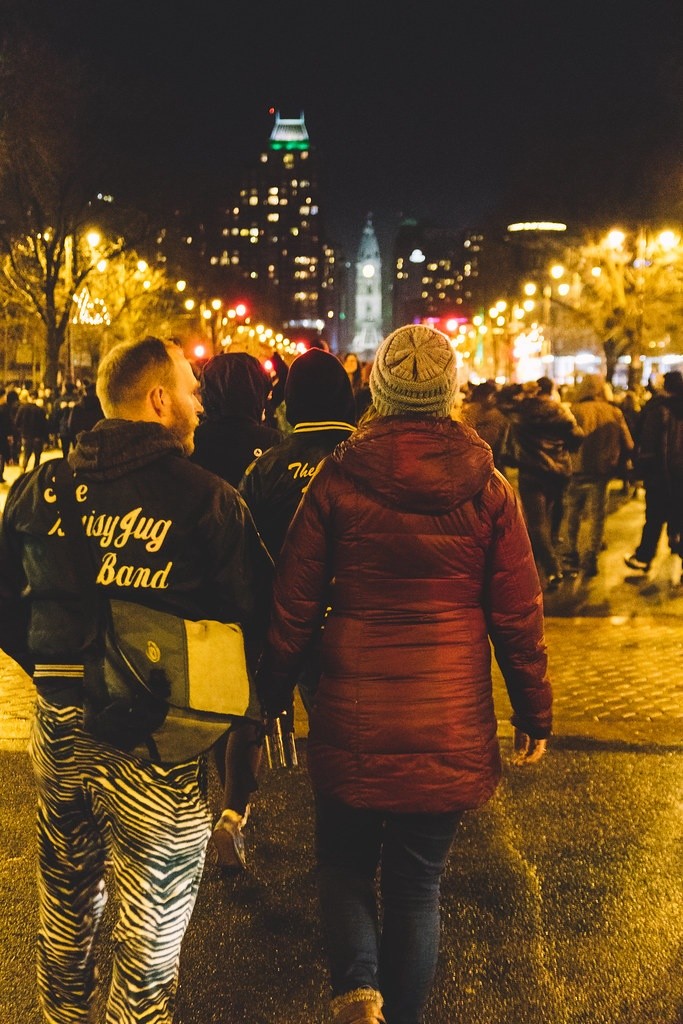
[335,1001,386,1024]
[543,539,682,591]
[213,811,249,869]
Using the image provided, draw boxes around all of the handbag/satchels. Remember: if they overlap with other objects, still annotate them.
[84,596,263,767]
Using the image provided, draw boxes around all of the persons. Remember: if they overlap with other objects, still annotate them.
[1,324,683,1024]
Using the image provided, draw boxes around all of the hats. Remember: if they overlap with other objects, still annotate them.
[368,324,459,421]
